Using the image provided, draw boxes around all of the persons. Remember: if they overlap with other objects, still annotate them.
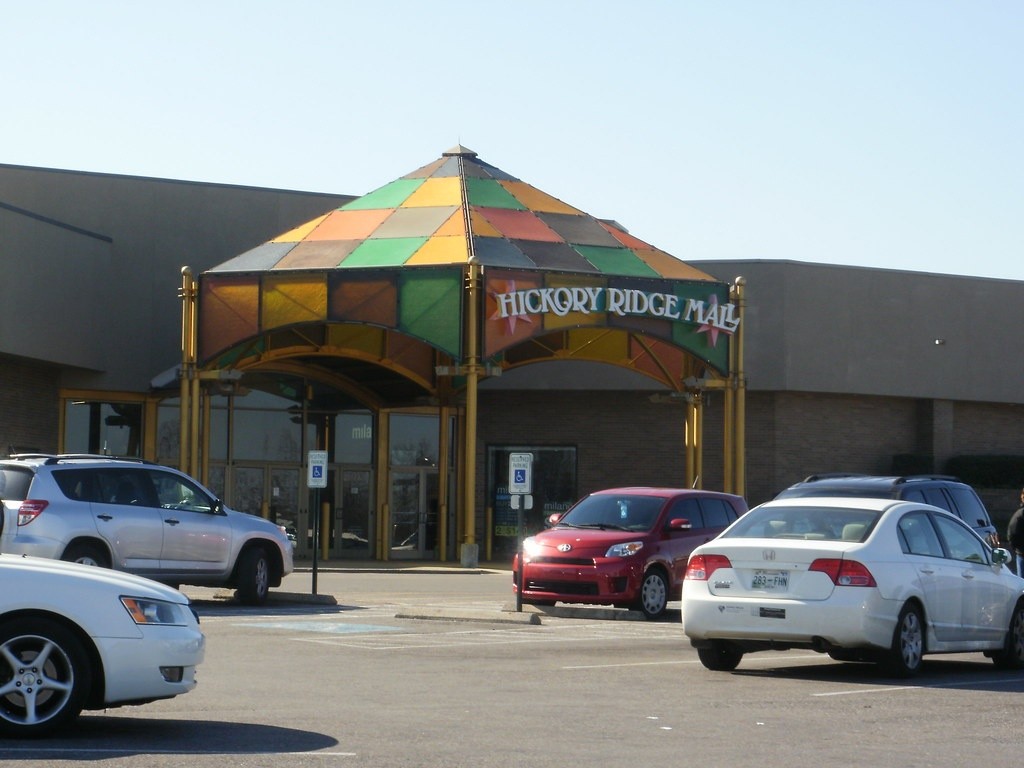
[1007,487,1024,580]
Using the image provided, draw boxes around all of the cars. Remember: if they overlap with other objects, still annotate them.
[0,554,206,738]
[513,476,749,619]
[682,498,1024,679]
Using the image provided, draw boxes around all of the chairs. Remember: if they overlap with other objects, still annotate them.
[764,521,792,538]
[842,522,868,540]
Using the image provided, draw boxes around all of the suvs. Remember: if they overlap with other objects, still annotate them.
[773,472,1000,550]
[0,453,294,603]
